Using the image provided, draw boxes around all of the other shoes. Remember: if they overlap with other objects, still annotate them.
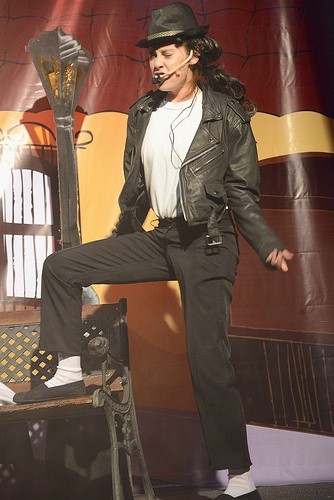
[13,380,88,403]
[215,490,262,500]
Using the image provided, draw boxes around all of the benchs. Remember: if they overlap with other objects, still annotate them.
[0,297,162,500]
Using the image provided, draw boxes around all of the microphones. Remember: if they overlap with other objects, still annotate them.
[152,50,193,84]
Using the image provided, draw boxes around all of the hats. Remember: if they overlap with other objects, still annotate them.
[135,3,209,48]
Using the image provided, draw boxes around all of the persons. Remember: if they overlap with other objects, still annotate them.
[12,1,294,500]
